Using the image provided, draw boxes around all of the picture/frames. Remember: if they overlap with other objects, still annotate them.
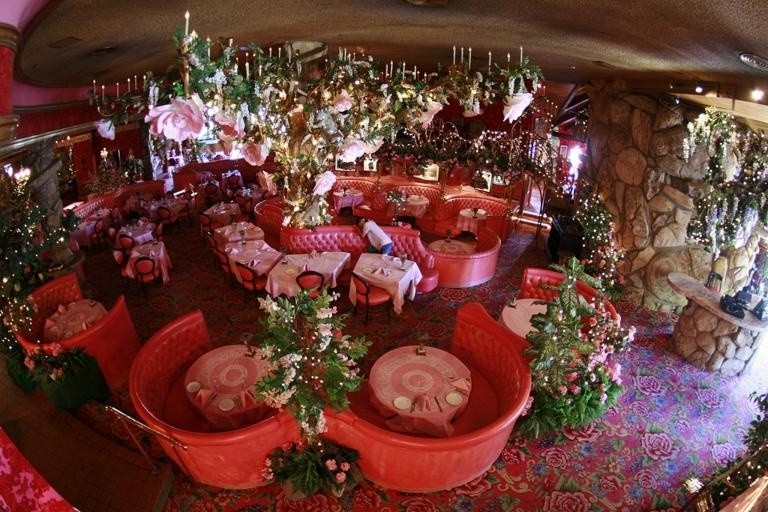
[559,144,568,159]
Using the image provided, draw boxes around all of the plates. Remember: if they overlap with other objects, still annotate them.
[393,257,402,263]
[391,392,464,415]
[363,267,373,275]
[285,269,296,275]
[320,251,333,259]
[185,379,236,413]
[42,300,97,342]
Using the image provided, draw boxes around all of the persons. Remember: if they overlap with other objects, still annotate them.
[312,166,337,197]
[354,215,393,255]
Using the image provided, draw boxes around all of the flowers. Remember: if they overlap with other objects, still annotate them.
[93,27,545,195]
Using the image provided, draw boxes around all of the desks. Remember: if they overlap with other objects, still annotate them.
[668,272,768,377]
